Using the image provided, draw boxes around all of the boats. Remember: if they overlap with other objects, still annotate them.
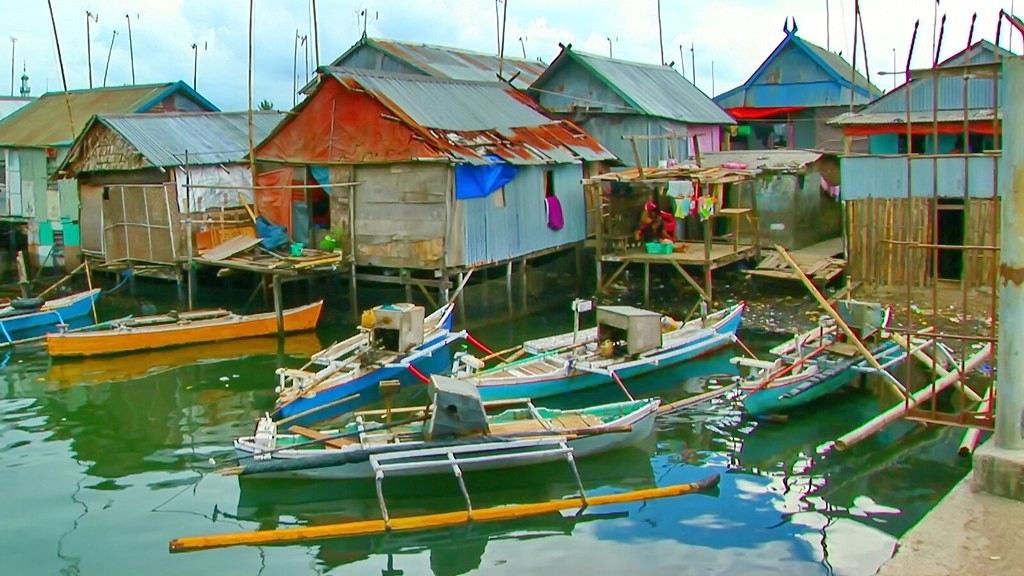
[232,373,661,479]
[0,288,102,335]
[45,332,320,391]
[449,298,747,407]
[46,299,324,356]
[740,300,901,416]
[275,302,458,430]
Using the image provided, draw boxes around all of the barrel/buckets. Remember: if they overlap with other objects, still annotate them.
[291,243,303,256]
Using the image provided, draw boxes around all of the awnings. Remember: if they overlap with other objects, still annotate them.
[825,110,1002,125]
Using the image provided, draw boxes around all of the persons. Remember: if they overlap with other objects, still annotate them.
[635,216,675,250]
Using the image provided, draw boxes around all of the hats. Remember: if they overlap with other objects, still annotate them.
[645,201,657,211]
